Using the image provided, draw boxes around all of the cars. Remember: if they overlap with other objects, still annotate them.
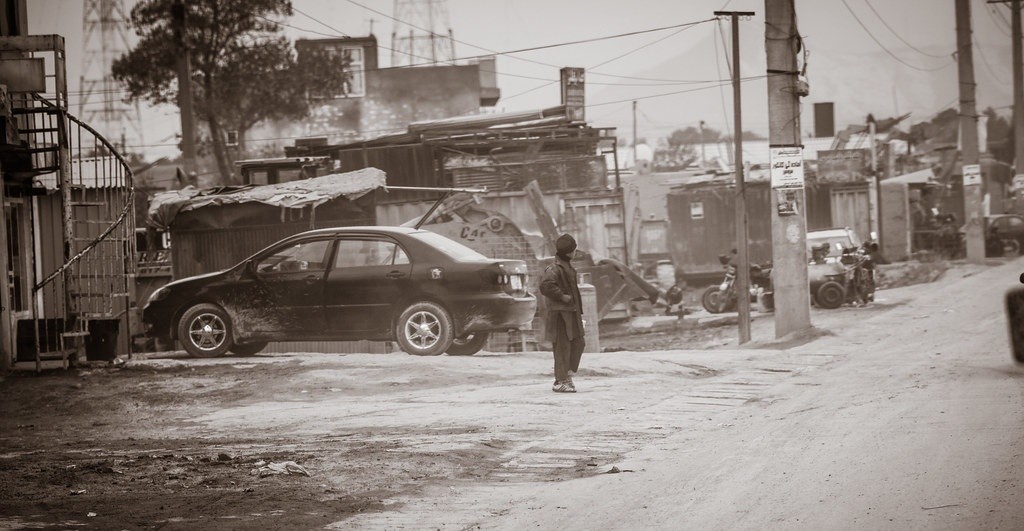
[956,214,1024,257]
[141,224,538,358]
[807,227,875,281]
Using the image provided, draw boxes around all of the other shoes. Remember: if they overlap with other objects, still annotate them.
[563,377,575,388]
[551,382,576,393]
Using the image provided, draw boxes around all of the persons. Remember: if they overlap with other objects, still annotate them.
[539,233,586,393]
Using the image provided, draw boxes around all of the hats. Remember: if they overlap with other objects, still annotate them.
[556,233,577,255]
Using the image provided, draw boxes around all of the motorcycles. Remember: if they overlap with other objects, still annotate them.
[702,242,848,315]
